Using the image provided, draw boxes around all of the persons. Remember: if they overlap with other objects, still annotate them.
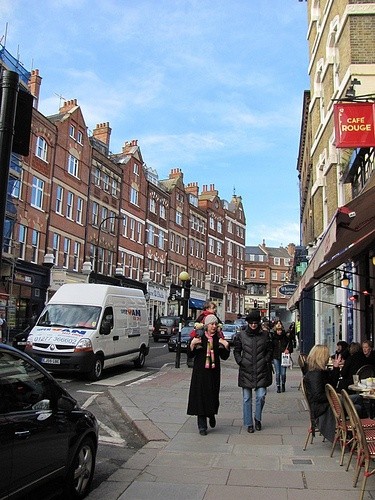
[303,340,375,443]
[260,314,300,393]
[187,314,230,436]
[233,310,273,433]
[0,316,6,344]
[194,301,224,350]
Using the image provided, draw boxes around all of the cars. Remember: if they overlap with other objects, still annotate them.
[0,342,99,500]
[222,318,245,345]
[168,326,195,352]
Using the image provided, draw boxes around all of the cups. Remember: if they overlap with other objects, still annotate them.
[361,377,375,386]
[353,375,359,387]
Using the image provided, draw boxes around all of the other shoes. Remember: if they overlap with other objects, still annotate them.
[281,383,285,392]
[277,386,281,393]
[247,425,255,433]
[209,416,216,428]
[199,429,208,435]
[254,417,262,431]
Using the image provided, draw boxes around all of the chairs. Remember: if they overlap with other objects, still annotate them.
[325,383,375,466]
[340,389,375,482]
[301,376,326,451]
[341,389,375,500]
[297,354,308,391]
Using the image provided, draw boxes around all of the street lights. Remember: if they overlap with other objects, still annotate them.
[94,217,125,273]
[176,272,190,369]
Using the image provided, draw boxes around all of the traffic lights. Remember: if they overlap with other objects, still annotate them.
[254,300,257,307]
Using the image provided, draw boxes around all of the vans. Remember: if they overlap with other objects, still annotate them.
[153,317,185,342]
[23,283,149,381]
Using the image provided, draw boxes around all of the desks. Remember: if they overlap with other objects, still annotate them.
[348,384,375,399]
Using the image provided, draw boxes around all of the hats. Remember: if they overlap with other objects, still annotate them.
[204,315,218,325]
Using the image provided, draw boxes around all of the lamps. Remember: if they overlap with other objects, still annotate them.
[308,244,315,247]
[351,78,362,86]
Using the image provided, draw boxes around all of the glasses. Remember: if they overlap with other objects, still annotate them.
[249,322,258,324]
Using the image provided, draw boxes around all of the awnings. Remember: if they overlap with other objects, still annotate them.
[285,184,375,313]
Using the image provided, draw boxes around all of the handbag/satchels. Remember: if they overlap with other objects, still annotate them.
[281,343,293,370]
